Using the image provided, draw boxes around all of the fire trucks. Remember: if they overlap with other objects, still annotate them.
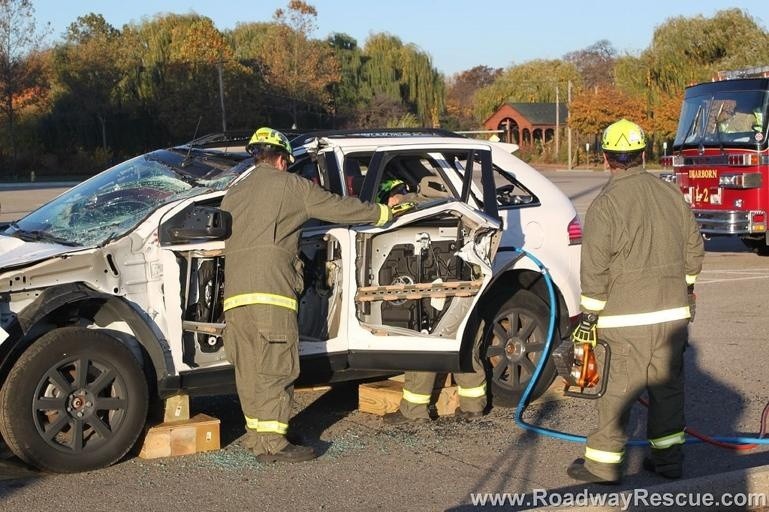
[656,64,769,258]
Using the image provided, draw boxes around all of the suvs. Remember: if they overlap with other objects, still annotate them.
[1,117,589,475]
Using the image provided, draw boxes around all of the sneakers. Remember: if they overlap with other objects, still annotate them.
[249,443,315,463]
[384,410,427,424]
[566,462,619,486]
[644,457,681,479]
[455,407,482,418]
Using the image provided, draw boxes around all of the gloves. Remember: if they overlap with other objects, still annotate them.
[393,200,418,216]
[570,314,597,348]
[688,292,697,322]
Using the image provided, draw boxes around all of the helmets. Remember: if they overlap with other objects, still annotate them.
[375,178,406,202]
[601,118,647,152]
[245,127,293,156]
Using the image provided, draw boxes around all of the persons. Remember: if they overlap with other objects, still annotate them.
[220,127,406,463]
[567,116,703,489]
